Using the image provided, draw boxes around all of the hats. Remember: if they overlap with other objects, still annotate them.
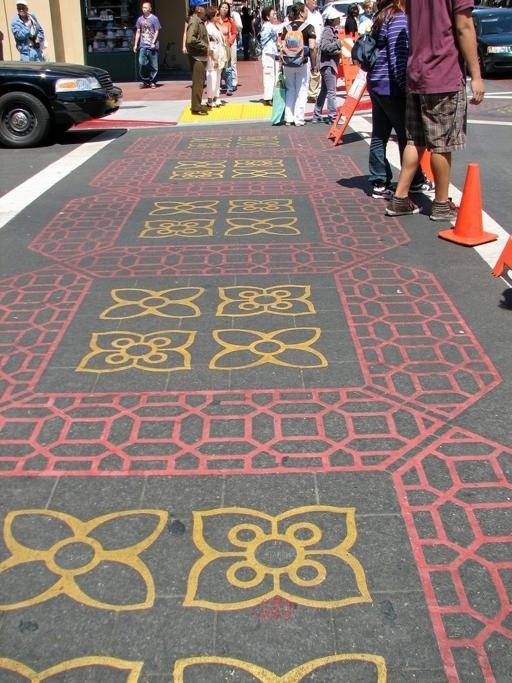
[322,7,345,25]
[190,0,209,7]
[16,0,28,7]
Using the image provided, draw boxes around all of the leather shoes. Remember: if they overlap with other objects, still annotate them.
[192,110,208,115]
[266,100,273,105]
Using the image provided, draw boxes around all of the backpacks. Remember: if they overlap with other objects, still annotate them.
[281,21,309,66]
[249,27,274,58]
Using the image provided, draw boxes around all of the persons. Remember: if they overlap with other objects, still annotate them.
[367,0,438,199]
[132,2,166,89]
[383,0,488,222]
[9,0,47,63]
[182,0,379,127]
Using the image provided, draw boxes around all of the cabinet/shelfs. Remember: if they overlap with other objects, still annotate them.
[84,5,136,52]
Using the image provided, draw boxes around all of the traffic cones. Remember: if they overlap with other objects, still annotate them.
[420,148,433,185]
[437,161,498,249]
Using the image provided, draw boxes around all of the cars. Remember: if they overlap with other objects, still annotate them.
[319,0,353,25]
[1,61,123,147]
[473,7,512,78]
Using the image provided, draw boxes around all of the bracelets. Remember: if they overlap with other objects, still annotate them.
[151,39,156,44]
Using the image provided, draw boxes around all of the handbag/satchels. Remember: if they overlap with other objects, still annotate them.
[40,33,48,48]
[352,35,380,72]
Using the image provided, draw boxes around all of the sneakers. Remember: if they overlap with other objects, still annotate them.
[213,101,222,107]
[286,115,344,127]
[207,102,216,107]
[220,85,236,95]
[150,82,156,89]
[430,197,460,220]
[372,185,395,200]
[139,82,149,87]
[385,196,424,216]
[410,178,436,192]
